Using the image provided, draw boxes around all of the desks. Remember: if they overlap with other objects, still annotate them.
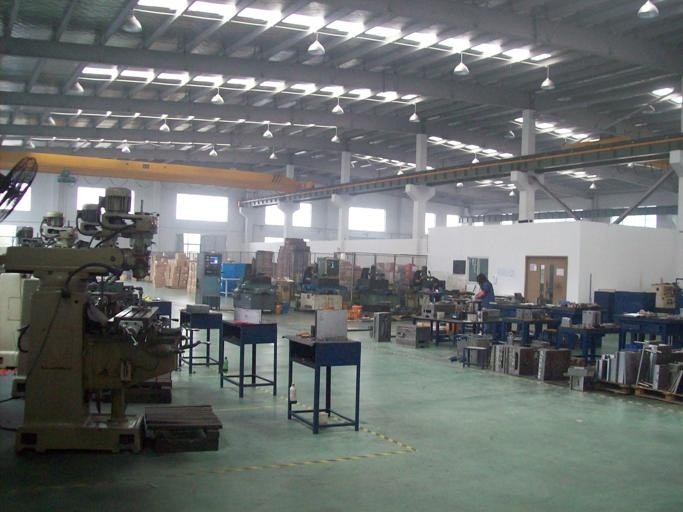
[380,292,682,402]
[179,307,223,375]
[283,333,361,433]
[217,318,279,400]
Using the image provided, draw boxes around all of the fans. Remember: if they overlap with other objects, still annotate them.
[0,158,40,227]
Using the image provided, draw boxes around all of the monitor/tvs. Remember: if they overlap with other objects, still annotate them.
[209,256,218,265]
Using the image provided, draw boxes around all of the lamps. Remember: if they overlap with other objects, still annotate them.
[20,1,682,173]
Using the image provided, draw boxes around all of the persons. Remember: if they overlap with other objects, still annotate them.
[303,267,317,284]
[473,273,495,309]
[413,271,423,286]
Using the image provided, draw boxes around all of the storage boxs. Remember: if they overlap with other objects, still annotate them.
[116,237,366,291]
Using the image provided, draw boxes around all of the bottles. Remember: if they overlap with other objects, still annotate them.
[223,356,228,369]
[142,295,161,303]
[290,383,297,402]
